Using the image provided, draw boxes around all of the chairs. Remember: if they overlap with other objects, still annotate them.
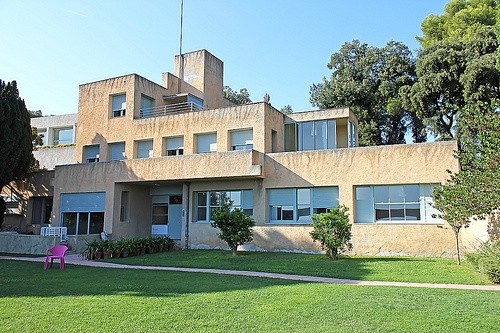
[42,245,69,270]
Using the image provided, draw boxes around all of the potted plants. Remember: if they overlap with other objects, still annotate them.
[79,233,177,261]
